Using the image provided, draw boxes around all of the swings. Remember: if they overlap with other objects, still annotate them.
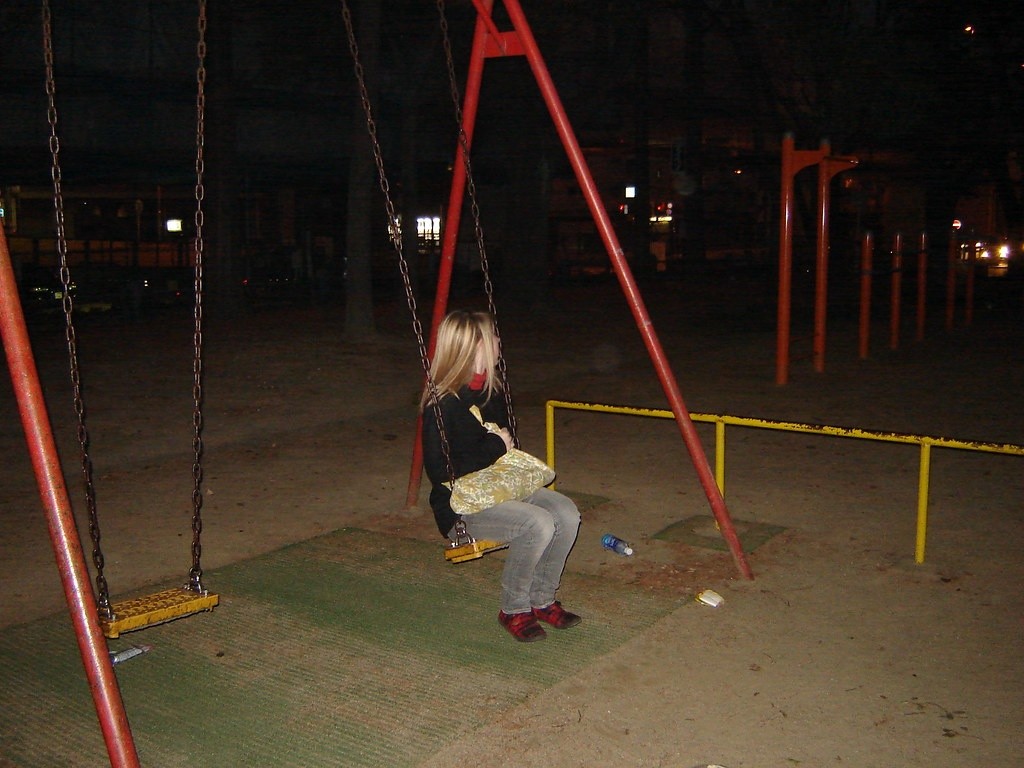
[339,1,522,563]
[36,1,218,639]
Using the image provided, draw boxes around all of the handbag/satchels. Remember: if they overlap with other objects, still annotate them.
[424,386,556,516]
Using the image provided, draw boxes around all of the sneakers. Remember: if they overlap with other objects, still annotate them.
[498,610,547,642]
[531,601,582,630]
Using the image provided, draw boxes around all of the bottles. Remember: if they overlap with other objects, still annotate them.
[601,534,632,556]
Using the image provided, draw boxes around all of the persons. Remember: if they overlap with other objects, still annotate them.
[419,308,582,642]
[10,251,22,281]
